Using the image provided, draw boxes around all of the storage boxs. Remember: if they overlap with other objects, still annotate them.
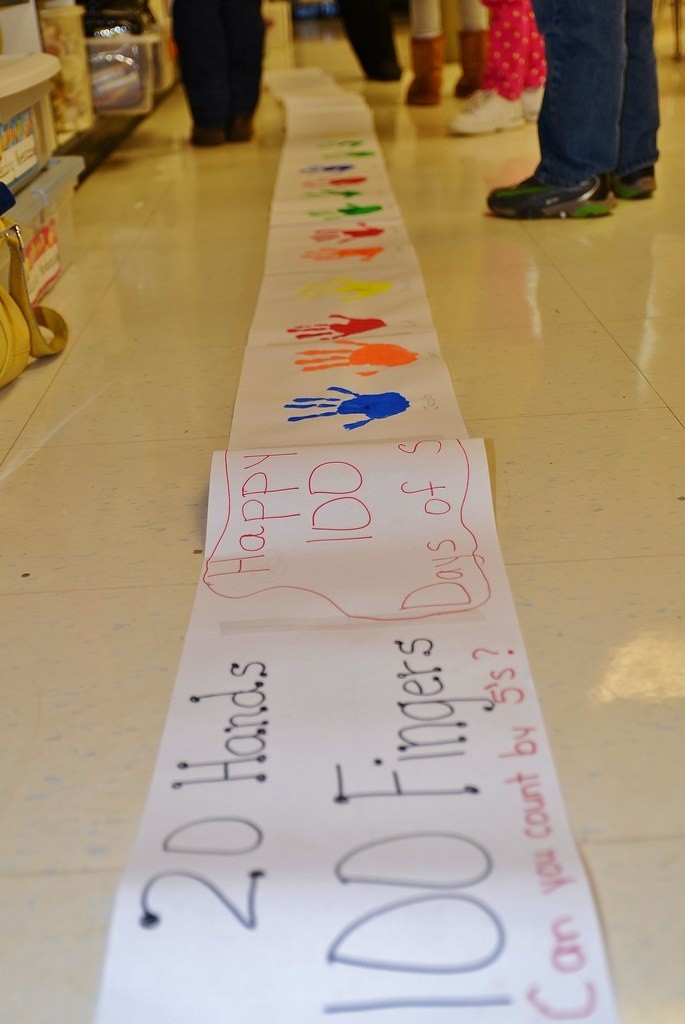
[2,157,87,305]
[0,53,61,193]
[86,33,162,119]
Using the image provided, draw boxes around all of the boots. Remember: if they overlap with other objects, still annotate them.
[454,29,492,97]
[408,34,444,106]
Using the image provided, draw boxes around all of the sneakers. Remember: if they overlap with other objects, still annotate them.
[450,88,526,136]
[522,81,546,120]
[613,166,657,198]
[488,170,616,218]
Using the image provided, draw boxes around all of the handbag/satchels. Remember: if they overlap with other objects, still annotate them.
[0,213,68,392]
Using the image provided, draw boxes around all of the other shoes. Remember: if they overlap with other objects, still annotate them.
[230,117,254,140]
[192,123,226,147]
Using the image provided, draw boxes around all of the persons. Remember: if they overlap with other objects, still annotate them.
[172,0,264,148]
[334,0,659,217]
